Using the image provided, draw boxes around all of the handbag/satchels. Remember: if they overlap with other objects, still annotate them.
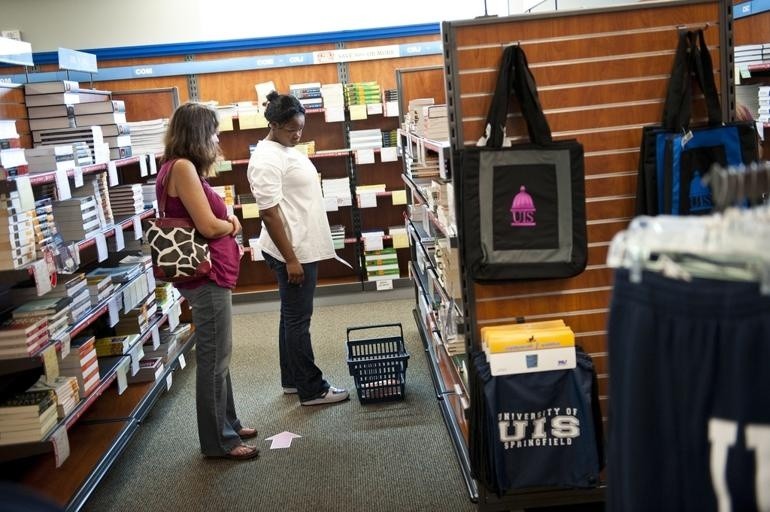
[452,47,587,287]
[635,23,763,216]
[141,216,212,283]
[469,344,607,498]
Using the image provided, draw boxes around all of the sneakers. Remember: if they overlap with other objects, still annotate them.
[284,387,349,407]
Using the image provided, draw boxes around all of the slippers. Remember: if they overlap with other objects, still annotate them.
[237,427,258,438]
[207,443,259,461]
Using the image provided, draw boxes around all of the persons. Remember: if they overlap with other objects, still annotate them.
[246,91,350,407]
[155,102,262,461]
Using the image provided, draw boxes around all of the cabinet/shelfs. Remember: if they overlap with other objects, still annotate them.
[1,58,192,512]
[397,67,482,492]
[158,48,411,293]
[729,11,770,155]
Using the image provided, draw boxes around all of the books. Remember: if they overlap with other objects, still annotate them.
[1,73,193,447]
[361,378,402,399]
[400,97,470,433]
[192,79,399,283]
[735,40,770,127]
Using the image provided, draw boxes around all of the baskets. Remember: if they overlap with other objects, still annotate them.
[347,322,409,404]
